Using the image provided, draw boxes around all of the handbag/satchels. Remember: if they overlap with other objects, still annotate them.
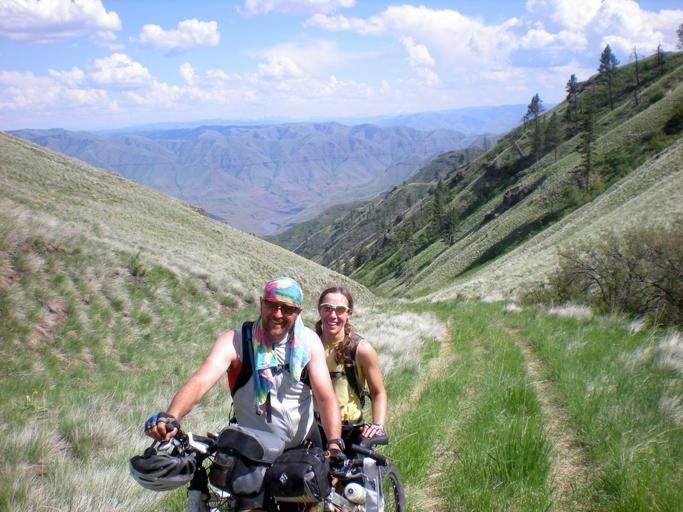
[208,422,272,499]
[261,446,334,507]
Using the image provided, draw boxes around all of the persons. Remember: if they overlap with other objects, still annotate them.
[300,286,388,488]
[143,276,347,512]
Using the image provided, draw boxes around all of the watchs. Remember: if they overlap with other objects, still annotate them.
[327,437,346,451]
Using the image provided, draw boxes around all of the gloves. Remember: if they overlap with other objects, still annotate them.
[142,411,180,433]
[359,421,385,435]
[327,448,350,477]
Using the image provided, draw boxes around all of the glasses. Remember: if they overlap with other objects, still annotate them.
[319,303,351,313]
[261,298,300,315]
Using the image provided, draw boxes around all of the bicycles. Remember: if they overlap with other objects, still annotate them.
[158,422,405,512]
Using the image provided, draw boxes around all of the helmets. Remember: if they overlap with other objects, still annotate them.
[126,448,201,492]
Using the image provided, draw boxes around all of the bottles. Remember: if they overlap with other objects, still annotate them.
[345,482,379,504]
